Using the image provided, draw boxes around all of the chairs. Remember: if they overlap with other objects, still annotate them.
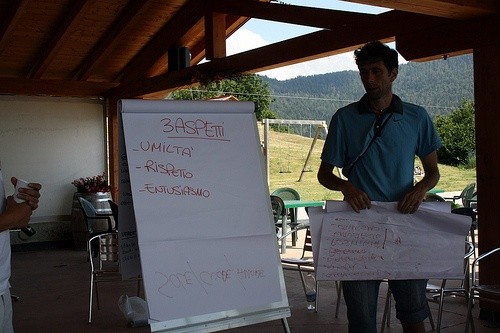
[78,197,118,262]
[87,233,123,324]
[270,184,500,333]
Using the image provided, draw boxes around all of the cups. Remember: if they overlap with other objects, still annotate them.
[13,179,33,204]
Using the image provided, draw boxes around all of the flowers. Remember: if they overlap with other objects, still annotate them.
[72,172,113,194]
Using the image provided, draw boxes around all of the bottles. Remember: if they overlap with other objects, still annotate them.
[305,272,316,310]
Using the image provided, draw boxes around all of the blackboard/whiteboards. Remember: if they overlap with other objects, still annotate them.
[121,111,291,333]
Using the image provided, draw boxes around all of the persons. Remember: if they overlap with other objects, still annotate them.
[317,41,442,333]
[0,161,42,333]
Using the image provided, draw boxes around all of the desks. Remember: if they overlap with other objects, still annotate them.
[281,200,324,255]
[427,189,444,195]
